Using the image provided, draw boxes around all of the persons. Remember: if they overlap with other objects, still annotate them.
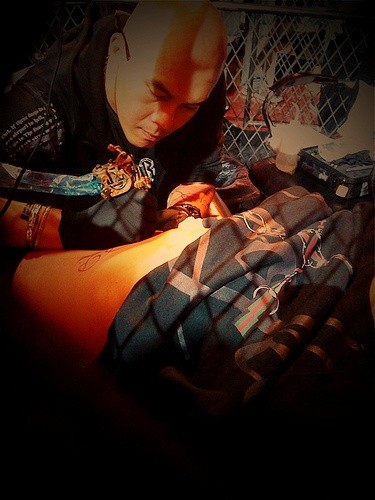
[0,1,228,251]
[0,186,375,470]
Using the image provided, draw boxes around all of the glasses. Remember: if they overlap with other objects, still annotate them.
[114,11,130,63]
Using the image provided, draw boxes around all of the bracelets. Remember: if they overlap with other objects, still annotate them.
[25,203,51,249]
